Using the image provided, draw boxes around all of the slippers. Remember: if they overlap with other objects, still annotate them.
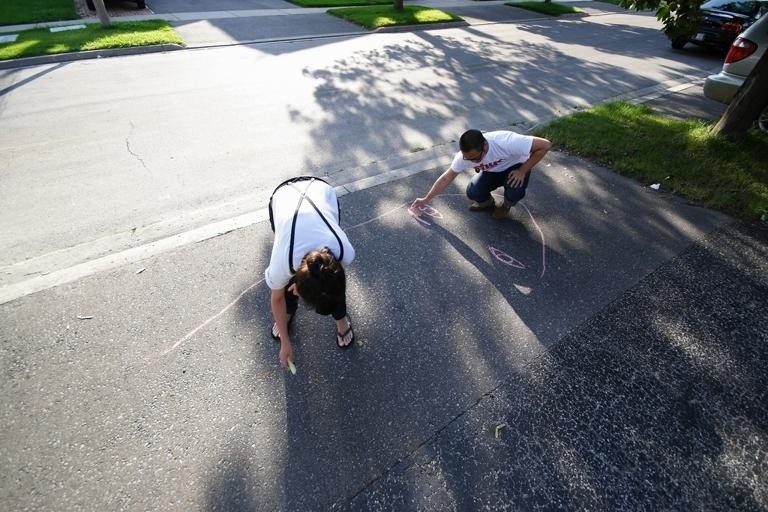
[335,313,354,348]
[271,312,294,340]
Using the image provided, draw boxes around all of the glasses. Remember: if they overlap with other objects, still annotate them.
[463,150,483,161]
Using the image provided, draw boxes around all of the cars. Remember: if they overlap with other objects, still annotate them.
[671,0,768,49]
[704,13,768,136]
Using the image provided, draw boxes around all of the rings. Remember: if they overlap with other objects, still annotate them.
[279,359,282,363]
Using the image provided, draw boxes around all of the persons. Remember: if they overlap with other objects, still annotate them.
[410,128,553,219]
[263,174,356,368]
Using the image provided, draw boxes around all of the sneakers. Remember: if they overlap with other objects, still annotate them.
[469,196,495,210]
[493,203,510,218]
